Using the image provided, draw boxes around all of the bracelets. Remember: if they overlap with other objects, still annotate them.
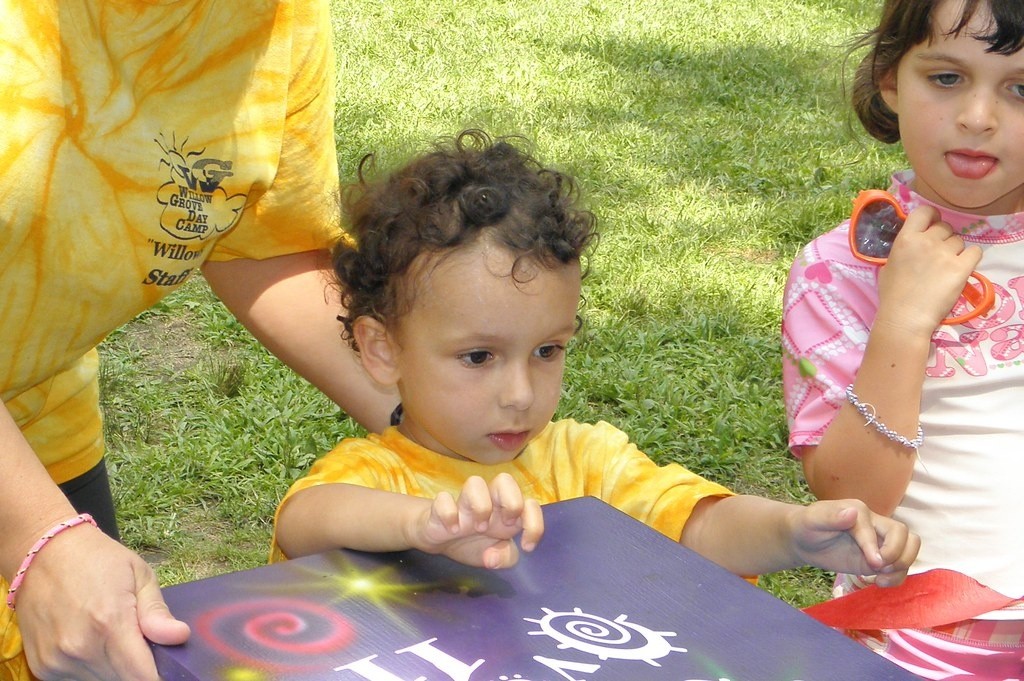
[8,512,103,610]
[848,382,924,452]
[390,403,404,428]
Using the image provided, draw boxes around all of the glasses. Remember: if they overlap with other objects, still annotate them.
[847,189,995,329]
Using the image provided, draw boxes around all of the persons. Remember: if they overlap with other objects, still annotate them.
[0,0,404,681]
[269,126,923,587]
[777,0,1024,681]
